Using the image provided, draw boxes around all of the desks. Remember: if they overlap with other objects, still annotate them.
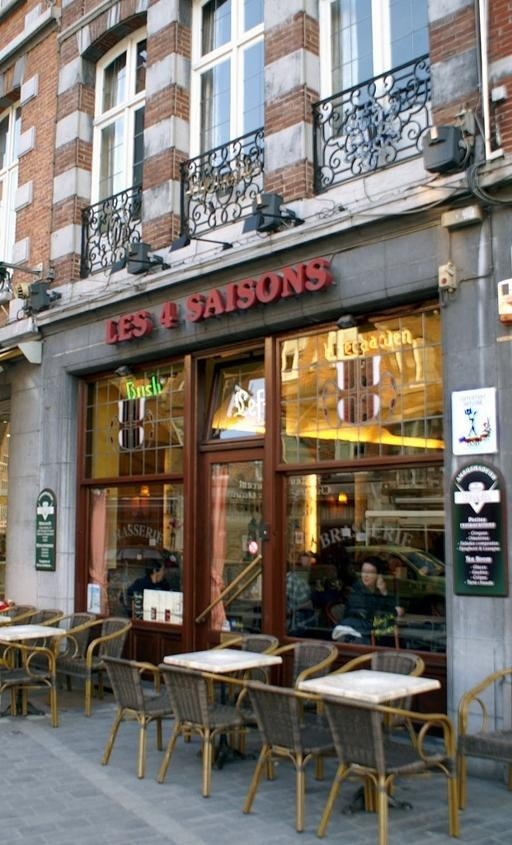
[392,613,445,649]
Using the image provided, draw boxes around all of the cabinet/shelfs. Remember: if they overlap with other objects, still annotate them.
[364,508,445,551]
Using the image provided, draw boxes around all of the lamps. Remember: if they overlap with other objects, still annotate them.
[169,233,231,253]
[110,256,172,275]
[242,211,304,233]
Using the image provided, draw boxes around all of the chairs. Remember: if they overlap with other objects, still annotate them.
[326,595,346,624]
[0,600,133,727]
[458,669,512,811]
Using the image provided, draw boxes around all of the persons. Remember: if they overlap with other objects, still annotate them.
[287,551,405,645]
[119,558,170,617]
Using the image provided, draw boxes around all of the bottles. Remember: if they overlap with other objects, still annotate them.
[150,606,156,620]
[165,608,170,622]
